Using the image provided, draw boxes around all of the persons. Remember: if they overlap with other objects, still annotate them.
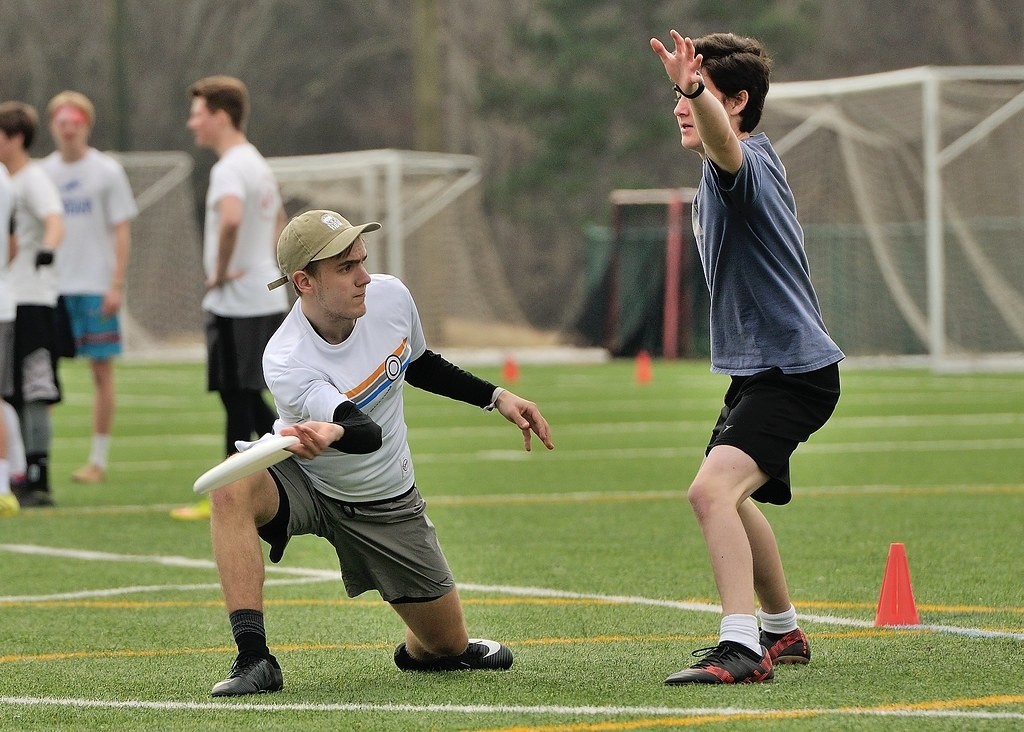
[210,209,552,697]
[37,90,139,476]
[0,101,66,508]
[174,76,289,519]
[646,30,845,685]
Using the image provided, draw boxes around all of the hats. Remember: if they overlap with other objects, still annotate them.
[267,210,382,291]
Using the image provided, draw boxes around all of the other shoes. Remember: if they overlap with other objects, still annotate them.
[171,498,211,520]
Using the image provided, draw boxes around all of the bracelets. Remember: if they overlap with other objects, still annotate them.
[674,70,705,98]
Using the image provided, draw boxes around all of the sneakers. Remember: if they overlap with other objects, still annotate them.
[16,492,54,508]
[663,640,775,686]
[758,626,812,665]
[212,650,283,697]
[395,637,513,671]
[72,460,106,484]
